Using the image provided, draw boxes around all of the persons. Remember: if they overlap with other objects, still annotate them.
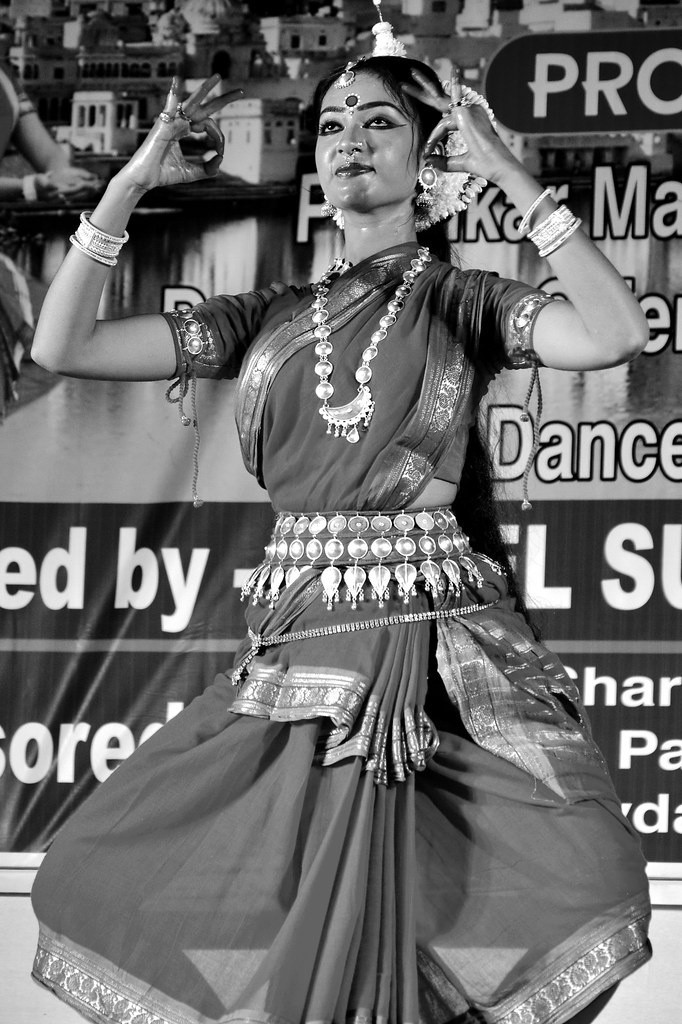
[30,56,652,1024]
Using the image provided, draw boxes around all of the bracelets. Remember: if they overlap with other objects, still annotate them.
[69,208,129,266]
[509,182,583,259]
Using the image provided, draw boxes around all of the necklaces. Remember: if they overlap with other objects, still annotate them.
[300,246,433,444]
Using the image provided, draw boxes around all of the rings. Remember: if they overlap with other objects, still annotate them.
[449,98,470,107]
[160,112,171,123]
[178,102,191,121]
